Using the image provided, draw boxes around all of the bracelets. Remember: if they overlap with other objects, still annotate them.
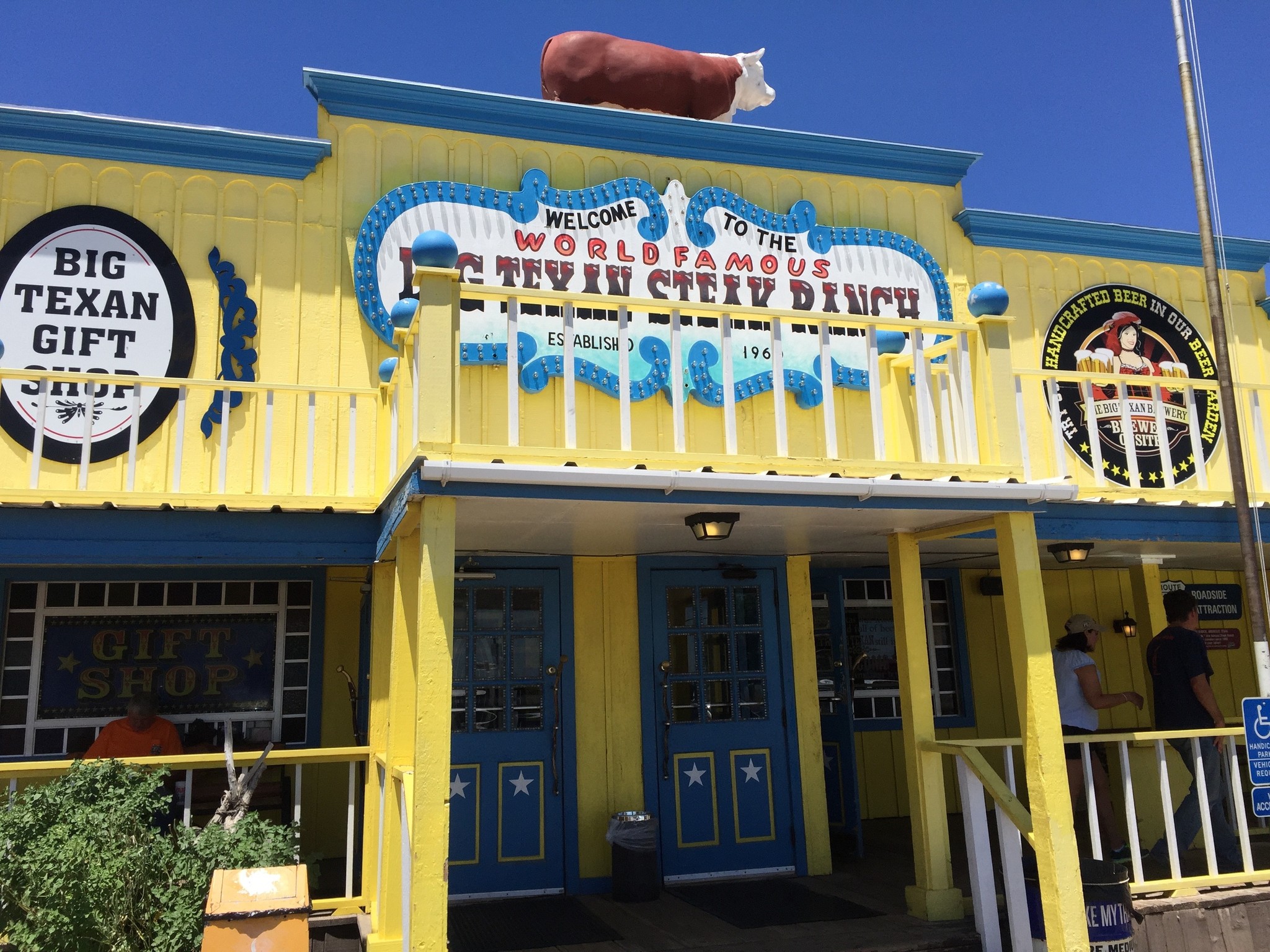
[1120,692,1128,703]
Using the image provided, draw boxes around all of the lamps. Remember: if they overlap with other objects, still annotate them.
[1113,610,1137,637]
[1047,542,1094,563]
[685,512,740,541]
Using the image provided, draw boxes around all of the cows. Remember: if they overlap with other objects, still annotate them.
[539,30,776,123]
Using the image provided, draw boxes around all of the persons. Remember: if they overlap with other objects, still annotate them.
[67,695,187,830]
[1145,590,1251,869]
[1054,613,1150,864]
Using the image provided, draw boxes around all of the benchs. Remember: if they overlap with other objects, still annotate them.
[62,741,292,843]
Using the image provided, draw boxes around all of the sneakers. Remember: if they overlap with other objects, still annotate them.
[1111,846,1149,863]
[1142,853,1185,871]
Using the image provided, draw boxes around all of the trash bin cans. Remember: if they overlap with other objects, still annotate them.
[605,809,660,905]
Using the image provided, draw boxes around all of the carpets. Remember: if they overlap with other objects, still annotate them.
[663,877,889,935]
[447,892,627,952]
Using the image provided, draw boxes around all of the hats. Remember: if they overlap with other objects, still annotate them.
[1064,613,1107,634]
